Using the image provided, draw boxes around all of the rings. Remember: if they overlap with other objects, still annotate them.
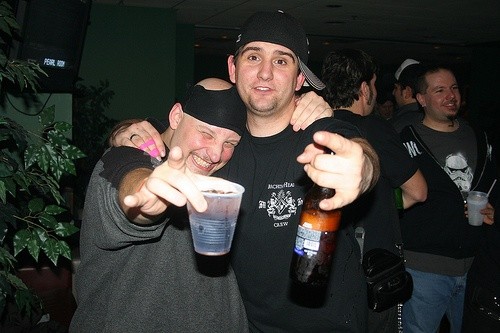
[130,134,140,141]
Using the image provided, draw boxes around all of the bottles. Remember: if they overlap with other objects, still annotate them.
[285,131,340,310]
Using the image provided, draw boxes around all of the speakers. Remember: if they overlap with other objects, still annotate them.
[2,0,93,93]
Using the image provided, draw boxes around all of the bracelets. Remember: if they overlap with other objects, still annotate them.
[135,177,148,192]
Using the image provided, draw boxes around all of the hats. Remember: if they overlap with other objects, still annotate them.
[234,9,327,90]
[393,59,427,85]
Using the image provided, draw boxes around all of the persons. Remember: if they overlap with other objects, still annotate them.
[387,62,494,333]
[103,11,380,332]
[70,78,246,333]
[319,46,427,333]
[377,59,424,125]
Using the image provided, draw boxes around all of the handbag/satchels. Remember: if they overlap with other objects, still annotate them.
[363,248,414,312]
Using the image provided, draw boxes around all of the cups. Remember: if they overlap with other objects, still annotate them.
[467,191,489,226]
[186,180,245,257]
